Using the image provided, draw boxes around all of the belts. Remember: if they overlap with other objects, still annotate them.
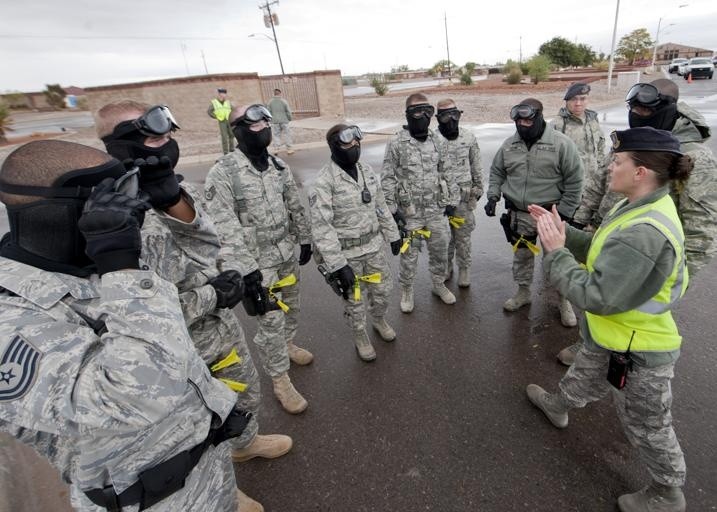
[339,225,381,249]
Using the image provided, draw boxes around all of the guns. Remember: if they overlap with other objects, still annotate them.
[318,265,342,297]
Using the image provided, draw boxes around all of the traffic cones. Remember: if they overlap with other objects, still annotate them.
[687,73,692,84]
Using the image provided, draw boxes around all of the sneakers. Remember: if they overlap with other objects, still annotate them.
[372,317,396,342]
[274,149,279,156]
[272,375,308,415]
[400,286,414,313]
[286,147,295,154]
[457,267,471,287]
[230,435,293,464]
[557,342,584,366]
[235,487,265,512]
[526,384,569,428]
[617,486,687,511]
[432,284,456,305]
[286,341,314,366]
[556,298,578,328]
[502,288,533,313]
[353,330,376,362]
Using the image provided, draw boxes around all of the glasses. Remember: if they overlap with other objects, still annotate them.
[510,104,534,120]
[409,105,434,118]
[113,166,142,200]
[437,109,461,122]
[624,83,660,107]
[245,104,273,123]
[337,125,363,144]
[134,105,180,137]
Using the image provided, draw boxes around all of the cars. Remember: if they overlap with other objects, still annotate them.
[677,62,688,75]
[667,57,688,73]
[683,58,714,80]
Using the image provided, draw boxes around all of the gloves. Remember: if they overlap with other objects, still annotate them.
[123,157,180,204]
[78,178,144,254]
[298,244,312,266]
[444,204,455,217]
[243,270,265,301]
[329,264,357,300]
[392,210,406,225]
[484,199,497,217]
[390,240,400,255]
[211,268,244,309]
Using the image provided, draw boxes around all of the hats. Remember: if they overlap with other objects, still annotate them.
[217,88,228,93]
[563,83,591,102]
[611,126,683,156]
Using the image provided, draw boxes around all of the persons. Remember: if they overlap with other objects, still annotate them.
[1,140,269,512]
[383,92,455,313]
[434,98,482,288]
[204,101,312,416]
[558,77,717,366]
[556,84,602,186]
[484,96,582,328]
[207,89,235,157]
[305,124,395,361]
[525,128,687,511]
[270,88,294,155]
[95,101,294,512]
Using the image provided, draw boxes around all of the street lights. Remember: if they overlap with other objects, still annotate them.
[247,32,285,75]
[650,4,688,70]
